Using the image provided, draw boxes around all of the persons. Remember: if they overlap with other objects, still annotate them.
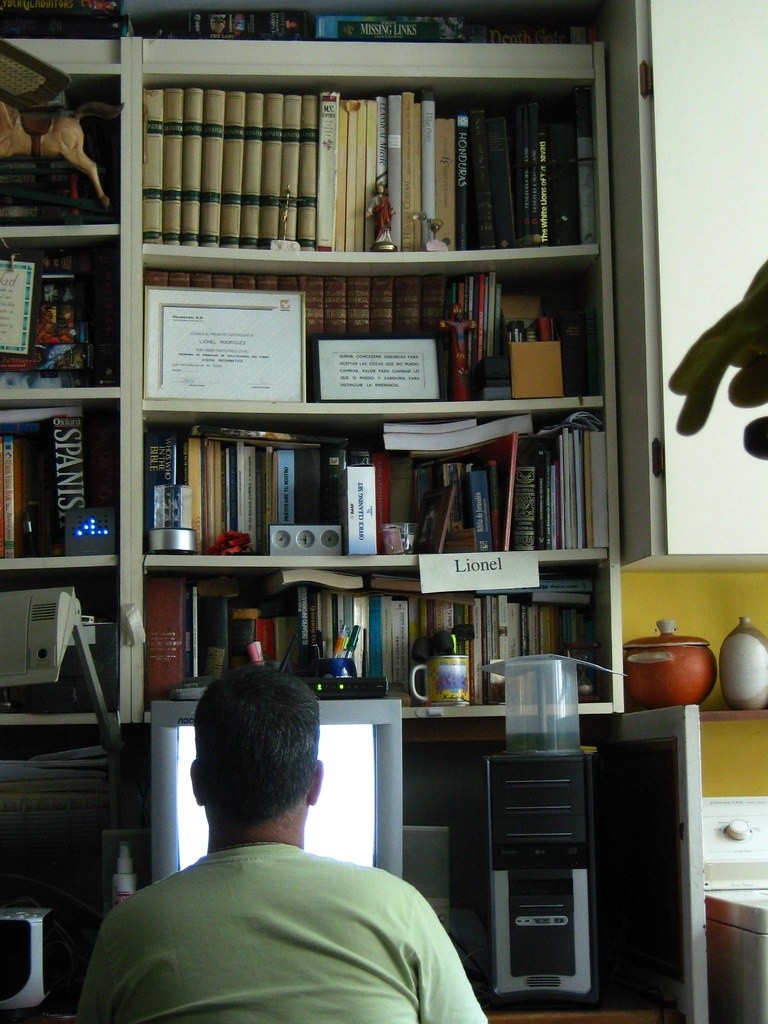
[365,184,396,244]
[78,667,490,1024]
[447,309,475,351]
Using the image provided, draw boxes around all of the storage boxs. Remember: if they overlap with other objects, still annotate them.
[510,342,563,399]
[341,465,377,556]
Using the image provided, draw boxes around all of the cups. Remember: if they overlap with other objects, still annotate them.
[248,660,294,674]
[410,655,469,706]
[381,522,417,554]
[319,658,357,678]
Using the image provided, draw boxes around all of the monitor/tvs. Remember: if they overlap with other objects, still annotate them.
[149,697,403,881]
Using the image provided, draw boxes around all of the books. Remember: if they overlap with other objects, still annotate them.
[146,270,601,398]
[0,87,113,221]
[0,408,111,558]
[142,412,610,556]
[142,86,592,249]
[0,1,597,43]
[144,571,592,703]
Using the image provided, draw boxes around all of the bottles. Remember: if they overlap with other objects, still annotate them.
[112,840,137,913]
[720,617,768,709]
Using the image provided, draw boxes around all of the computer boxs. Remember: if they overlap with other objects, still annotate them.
[486,750,602,1014]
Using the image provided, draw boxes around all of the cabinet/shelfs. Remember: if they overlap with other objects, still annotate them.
[0,0,768,722]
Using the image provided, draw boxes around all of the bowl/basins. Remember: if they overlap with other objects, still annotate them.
[623,620,717,708]
